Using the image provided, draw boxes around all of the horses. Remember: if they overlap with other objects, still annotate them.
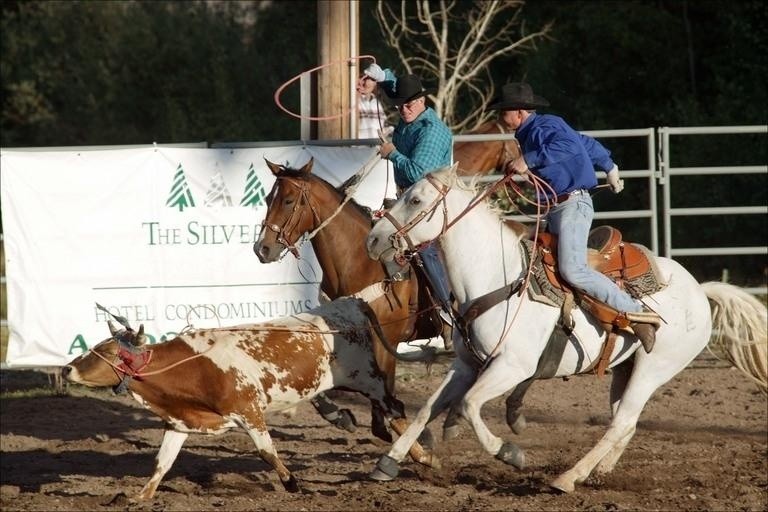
[452,118,528,177]
[364,159,768,494]
[252,156,544,445]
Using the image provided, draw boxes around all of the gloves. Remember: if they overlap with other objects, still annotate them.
[364,64,385,83]
[606,164,624,193]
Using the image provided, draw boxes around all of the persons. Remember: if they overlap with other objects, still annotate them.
[365,63,461,350]
[358,64,388,138]
[483,81,655,354]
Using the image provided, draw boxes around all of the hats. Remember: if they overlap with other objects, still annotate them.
[484,82,550,112]
[382,74,437,104]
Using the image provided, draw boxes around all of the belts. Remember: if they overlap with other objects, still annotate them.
[545,189,587,210]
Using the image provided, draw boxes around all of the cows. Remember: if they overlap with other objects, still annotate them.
[61,295,458,505]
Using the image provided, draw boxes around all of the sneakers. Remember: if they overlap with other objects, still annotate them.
[631,305,655,353]
